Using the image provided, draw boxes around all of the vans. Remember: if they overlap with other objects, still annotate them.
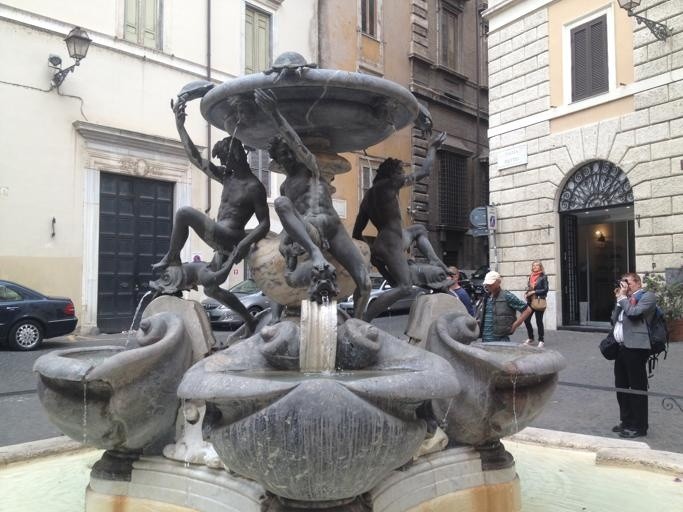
[337,272,411,318]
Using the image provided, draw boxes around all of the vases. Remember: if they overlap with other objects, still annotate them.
[664,319,683,342]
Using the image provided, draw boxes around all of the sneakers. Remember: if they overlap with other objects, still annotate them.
[525,338,534,344]
[612,422,647,438]
[538,341,545,348]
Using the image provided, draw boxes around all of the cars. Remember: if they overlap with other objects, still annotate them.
[0,278,78,351]
[454,270,472,300]
[470,264,489,296]
[199,277,272,331]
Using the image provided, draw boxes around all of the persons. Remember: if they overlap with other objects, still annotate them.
[146,99,270,342]
[268,135,369,320]
[443,264,474,318]
[521,259,547,348]
[472,269,531,344]
[609,274,656,438]
[350,130,453,324]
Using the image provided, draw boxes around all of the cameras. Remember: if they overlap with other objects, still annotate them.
[612,279,628,288]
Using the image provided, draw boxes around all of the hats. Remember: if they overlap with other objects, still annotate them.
[482,271,501,285]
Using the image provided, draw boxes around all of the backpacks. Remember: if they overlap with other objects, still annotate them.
[636,292,667,355]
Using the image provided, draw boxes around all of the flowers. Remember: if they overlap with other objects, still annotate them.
[641,262,683,320]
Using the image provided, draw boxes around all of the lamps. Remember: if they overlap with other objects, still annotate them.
[595,231,608,245]
[48,27,92,87]
[617,0,668,41]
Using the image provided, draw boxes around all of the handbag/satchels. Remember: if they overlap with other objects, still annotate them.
[531,299,547,312]
[599,333,620,360]
